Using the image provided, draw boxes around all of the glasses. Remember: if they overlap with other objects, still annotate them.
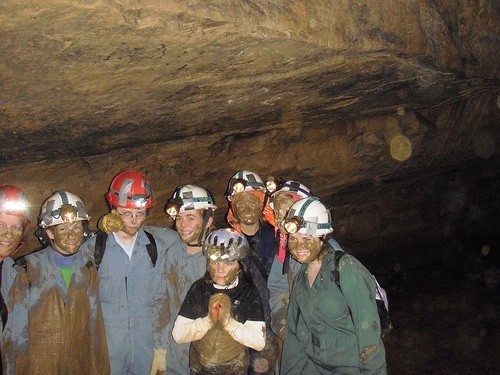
[115,207,145,218]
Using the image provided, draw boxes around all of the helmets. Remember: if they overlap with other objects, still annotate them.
[0,185,31,221]
[202,229,250,262]
[282,197,334,236]
[172,184,217,211]
[271,180,311,202]
[37,190,90,226]
[226,169,267,201]
[106,170,156,208]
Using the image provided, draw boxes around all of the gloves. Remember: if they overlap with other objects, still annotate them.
[97,209,124,234]
[150,349,167,374]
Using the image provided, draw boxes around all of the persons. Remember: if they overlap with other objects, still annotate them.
[150,184,278,375]
[171,227,266,375]
[280,196,394,375]
[0,170,345,375]
[0,185,30,375]
[77,170,180,375]
[15,190,123,375]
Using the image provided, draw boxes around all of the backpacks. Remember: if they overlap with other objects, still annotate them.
[331,250,393,339]
